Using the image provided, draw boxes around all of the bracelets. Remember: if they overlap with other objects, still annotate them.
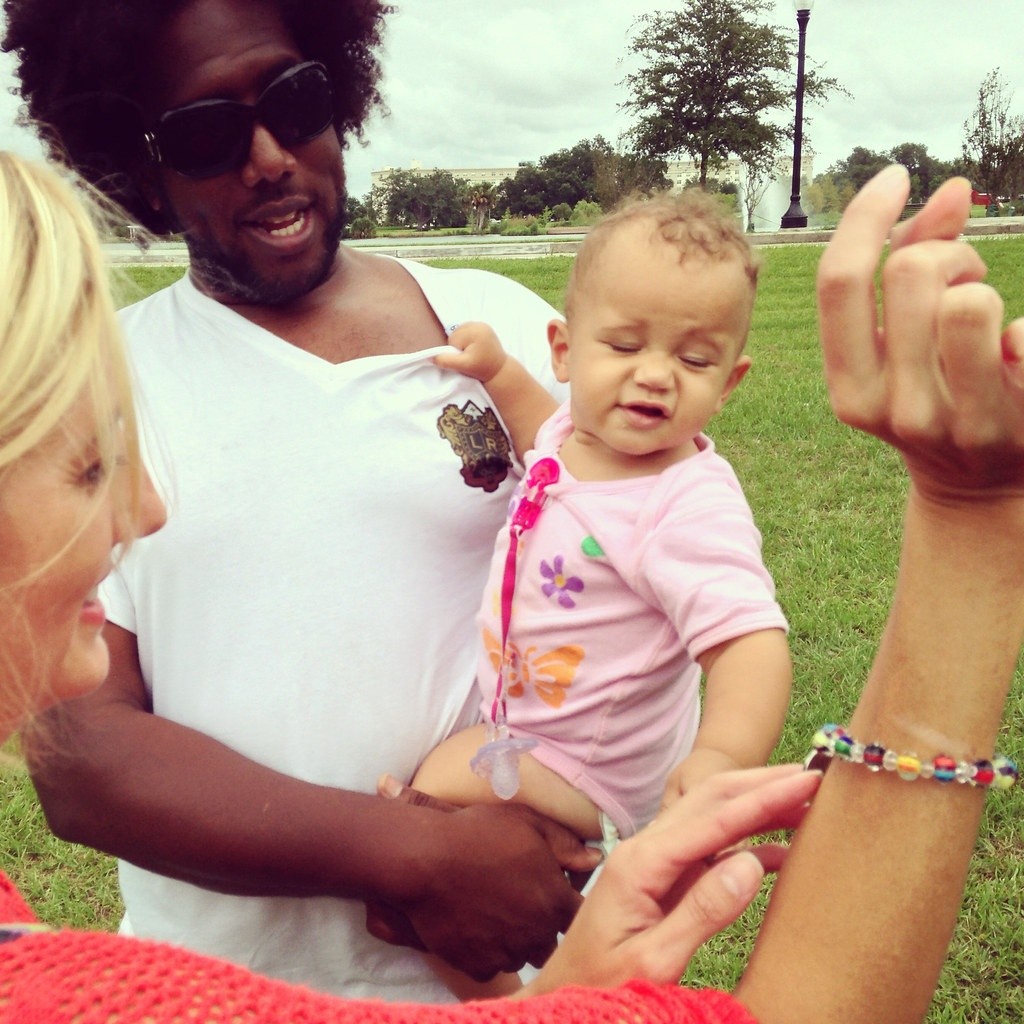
[813,725,1018,787]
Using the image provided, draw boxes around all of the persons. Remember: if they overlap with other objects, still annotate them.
[411,195,792,1001]
[1,0,602,1007]
[1,165,1024,1024]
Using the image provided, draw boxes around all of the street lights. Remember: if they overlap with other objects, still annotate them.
[781,0,815,229]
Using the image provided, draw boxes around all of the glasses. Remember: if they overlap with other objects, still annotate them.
[135,60,336,180]
